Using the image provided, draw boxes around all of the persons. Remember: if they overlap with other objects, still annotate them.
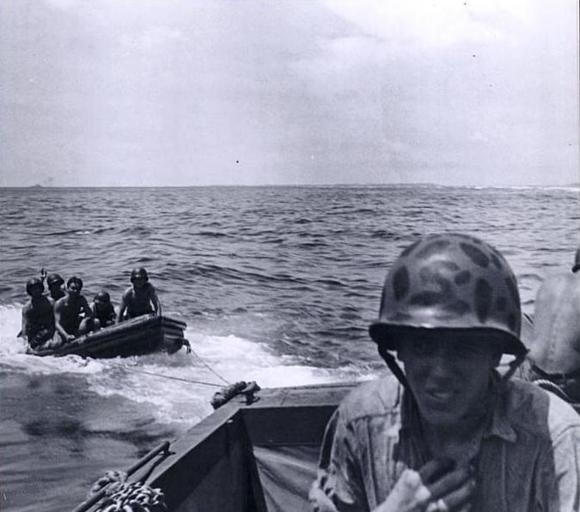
[21,278,56,353]
[525,248,579,408]
[88,291,117,330]
[51,276,100,350]
[40,268,69,306]
[304,232,579,510]
[114,267,161,324]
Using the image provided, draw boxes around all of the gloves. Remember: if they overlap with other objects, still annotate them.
[26,313,187,360]
[70,379,372,511]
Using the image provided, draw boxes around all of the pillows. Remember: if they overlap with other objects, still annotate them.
[93,292,110,305]
[47,274,64,290]
[131,267,147,283]
[26,279,44,296]
[367,234,528,355]
